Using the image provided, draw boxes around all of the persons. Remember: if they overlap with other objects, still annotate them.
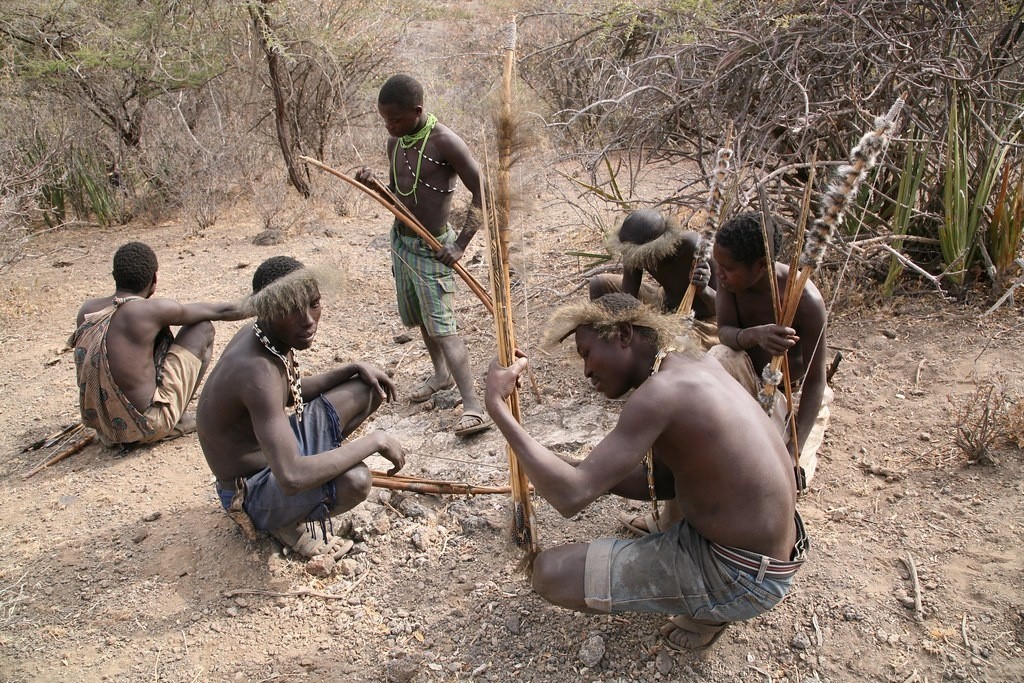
[486,294,809,651]
[68,242,255,442]
[353,75,493,435]
[587,210,719,348]
[198,256,406,560]
[706,212,834,489]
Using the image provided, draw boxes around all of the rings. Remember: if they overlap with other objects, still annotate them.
[700,275,702,280]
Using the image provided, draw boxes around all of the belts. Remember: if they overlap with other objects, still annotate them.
[393,218,447,237]
[707,541,805,579]
[778,377,804,391]
[216,480,247,491]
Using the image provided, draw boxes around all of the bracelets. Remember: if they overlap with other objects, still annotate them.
[736,330,746,350]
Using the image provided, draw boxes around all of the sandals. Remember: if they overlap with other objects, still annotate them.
[162,412,198,440]
[664,613,732,650]
[408,375,456,402]
[617,512,660,537]
[454,407,495,435]
[268,521,353,559]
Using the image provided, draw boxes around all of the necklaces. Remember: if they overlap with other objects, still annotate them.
[392,113,437,197]
[645,347,675,522]
[253,324,303,421]
